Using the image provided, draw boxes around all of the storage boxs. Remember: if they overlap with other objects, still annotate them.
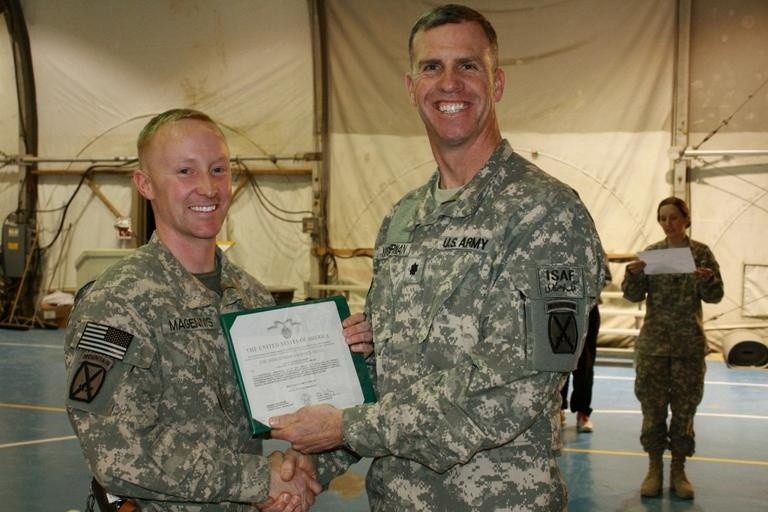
[40,297,76,329]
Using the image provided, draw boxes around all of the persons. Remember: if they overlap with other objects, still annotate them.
[268,3,613,511]
[62,107,377,512]
[560,299,602,431]
[618,197,726,503]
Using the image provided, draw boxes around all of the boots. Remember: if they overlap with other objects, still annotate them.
[559,410,567,427]
[576,411,593,433]
[637,447,664,499]
[667,453,697,503]
